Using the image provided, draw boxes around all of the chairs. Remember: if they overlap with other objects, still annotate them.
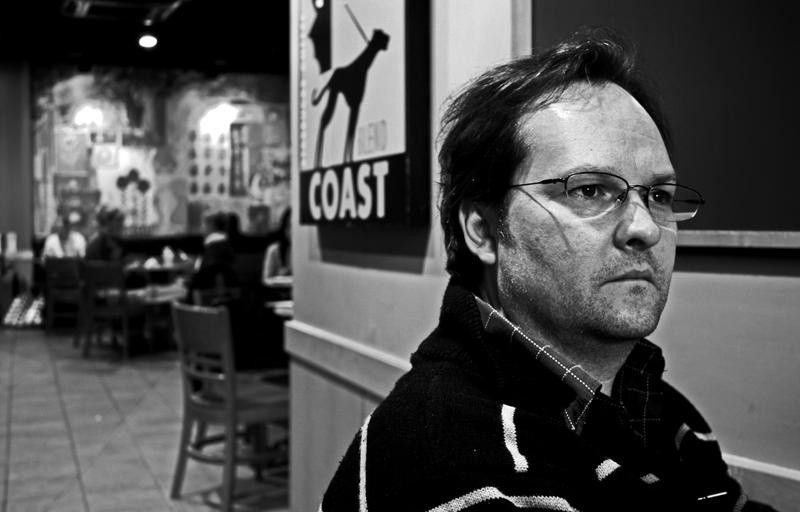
[44,255,84,335]
[73,261,131,359]
[169,298,289,512]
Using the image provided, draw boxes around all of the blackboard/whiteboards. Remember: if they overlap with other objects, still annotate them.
[511,0,800,249]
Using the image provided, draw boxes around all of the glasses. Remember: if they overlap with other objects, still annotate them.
[509,171,706,224]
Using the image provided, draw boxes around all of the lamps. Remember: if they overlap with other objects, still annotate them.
[135,9,165,48]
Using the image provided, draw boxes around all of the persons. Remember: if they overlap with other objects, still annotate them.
[85,205,125,264]
[38,215,87,267]
[320,22,780,512]
[190,206,294,300]
